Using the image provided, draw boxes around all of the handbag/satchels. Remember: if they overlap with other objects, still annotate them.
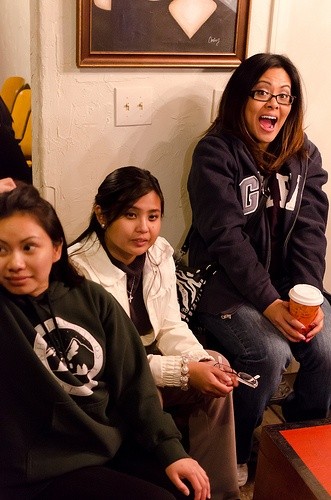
[173,257,210,325]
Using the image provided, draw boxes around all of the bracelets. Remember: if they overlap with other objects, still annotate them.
[182,357,189,392]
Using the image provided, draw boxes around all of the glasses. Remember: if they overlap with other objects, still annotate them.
[213,362,261,388]
[248,89,296,106]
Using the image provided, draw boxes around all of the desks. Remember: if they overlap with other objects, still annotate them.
[253,418,331,500]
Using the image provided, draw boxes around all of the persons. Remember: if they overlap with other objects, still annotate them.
[186,52,331,485]
[0,95,34,183]
[68,166,242,500]
[0,177,212,500]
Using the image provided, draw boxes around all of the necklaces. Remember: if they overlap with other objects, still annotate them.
[125,275,136,304]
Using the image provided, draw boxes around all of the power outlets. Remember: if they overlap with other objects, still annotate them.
[210,88,225,123]
[114,85,152,126]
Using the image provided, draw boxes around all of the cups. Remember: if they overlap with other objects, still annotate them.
[289,284,324,335]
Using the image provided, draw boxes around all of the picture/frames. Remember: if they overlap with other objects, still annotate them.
[76,0,252,68]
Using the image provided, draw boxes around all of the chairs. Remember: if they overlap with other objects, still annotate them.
[0,75,32,167]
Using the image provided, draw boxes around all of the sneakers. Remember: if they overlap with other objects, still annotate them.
[237,463,248,485]
